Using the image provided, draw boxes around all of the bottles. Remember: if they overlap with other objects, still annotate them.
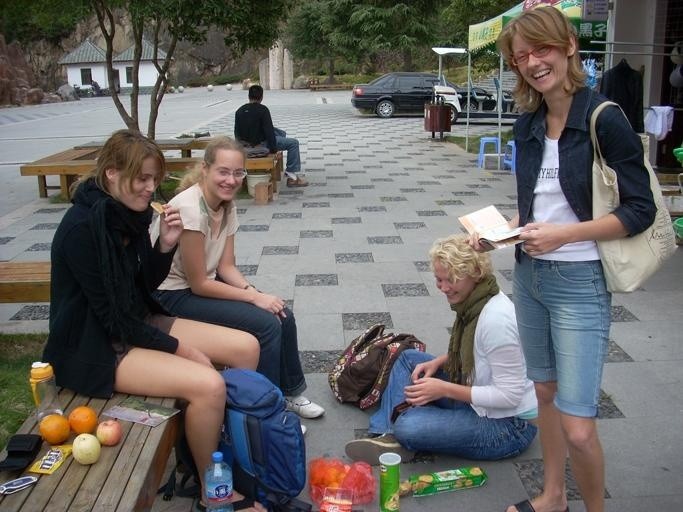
[29,361,64,426]
[203,451,235,512]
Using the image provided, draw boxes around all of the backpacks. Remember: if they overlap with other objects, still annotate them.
[174,366,305,507]
[328,324,426,410]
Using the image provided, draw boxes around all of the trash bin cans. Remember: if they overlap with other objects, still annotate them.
[423,102,451,134]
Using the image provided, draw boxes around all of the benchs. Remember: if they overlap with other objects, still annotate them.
[310,83,352,91]
[21,139,284,201]
[0,261,51,303]
[1,386,182,511]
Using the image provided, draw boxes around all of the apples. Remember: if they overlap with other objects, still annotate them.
[96,420,121,447]
[72,433,101,465]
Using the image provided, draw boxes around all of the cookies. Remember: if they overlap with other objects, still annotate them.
[399,479,412,496]
[150,201,165,215]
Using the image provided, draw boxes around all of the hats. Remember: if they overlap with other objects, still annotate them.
[669,42,683,88]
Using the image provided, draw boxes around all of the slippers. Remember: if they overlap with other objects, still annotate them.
[503,500,569,512]
[197,492,272,512]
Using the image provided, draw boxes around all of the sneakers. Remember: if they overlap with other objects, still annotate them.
[287,177,308,186]
[283,395,325,419]
[345,432,415,466]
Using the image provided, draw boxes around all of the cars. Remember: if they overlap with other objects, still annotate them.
[351,72,496,118]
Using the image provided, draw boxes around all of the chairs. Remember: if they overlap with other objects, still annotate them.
[442,75,462,100]
[469,78,489,113]
[494,77,514,113]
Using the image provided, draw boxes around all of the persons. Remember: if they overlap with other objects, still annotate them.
[47,129,270,512]
[234,85,310,188]
[343,234,540,468]
[90,80,102,97]
[148,134,327,435]
[462,6,657,512]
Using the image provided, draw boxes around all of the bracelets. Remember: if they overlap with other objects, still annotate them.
[243,281,257,293]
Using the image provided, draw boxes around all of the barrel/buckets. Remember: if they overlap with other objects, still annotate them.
[246,173,273,197]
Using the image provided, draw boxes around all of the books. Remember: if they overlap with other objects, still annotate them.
[457,201,528,252]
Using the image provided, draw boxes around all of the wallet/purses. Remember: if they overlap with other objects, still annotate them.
[391,402,411,424]
[0,434,42,470]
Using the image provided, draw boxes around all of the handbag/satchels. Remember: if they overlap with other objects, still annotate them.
[592,134,678,293]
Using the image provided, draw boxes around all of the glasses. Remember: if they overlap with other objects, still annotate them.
[208,163,247,179]
[512,45,551,66]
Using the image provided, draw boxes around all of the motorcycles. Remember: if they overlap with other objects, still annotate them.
[87,87,109,98]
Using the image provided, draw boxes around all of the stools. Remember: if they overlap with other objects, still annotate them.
[503,139,518,174]
[478,137,499,169]
